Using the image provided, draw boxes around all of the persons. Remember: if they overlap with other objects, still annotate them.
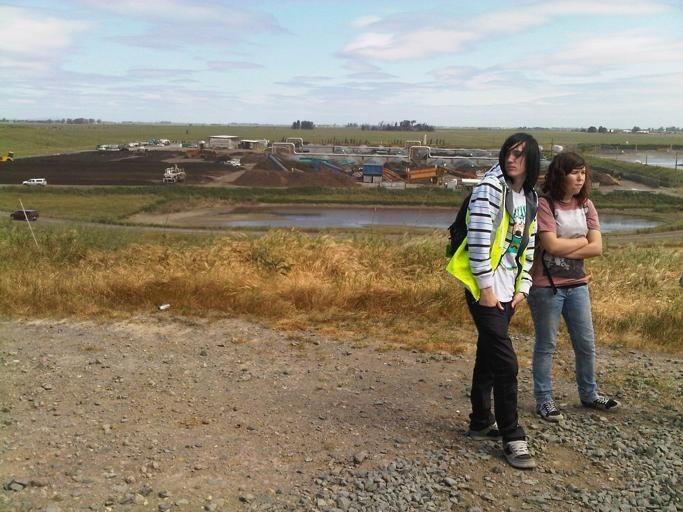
[525,151,621,423]
[444,129,537,471]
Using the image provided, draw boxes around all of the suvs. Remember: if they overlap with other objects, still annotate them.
[8,209,41,222]
[23,178,47,187]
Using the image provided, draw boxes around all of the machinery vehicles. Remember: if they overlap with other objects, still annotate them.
[96,138,169,153]
[403,144,441,185]
[0,150,15,168]
[223,158,241,168]
[161,164,186,185]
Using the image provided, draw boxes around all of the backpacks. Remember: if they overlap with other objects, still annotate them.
[448,192,472,256]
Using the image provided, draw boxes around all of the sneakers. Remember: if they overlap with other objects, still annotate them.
[581,396,623,413]
[536,400,564,423]
[468,423,506,441]
[503,440,536,469]
